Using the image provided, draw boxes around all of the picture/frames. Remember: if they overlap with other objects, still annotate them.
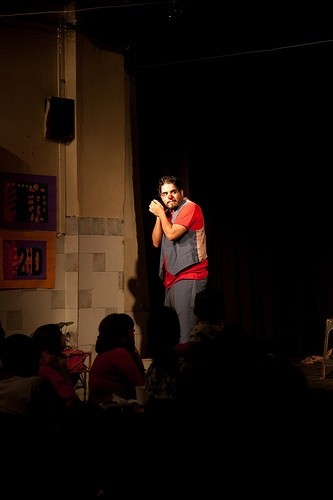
[0,171,57,231]
[0,231,56,289]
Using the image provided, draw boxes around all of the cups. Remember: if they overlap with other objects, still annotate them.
[136,386,147,402]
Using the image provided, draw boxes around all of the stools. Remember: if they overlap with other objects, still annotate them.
[319,318,333,379]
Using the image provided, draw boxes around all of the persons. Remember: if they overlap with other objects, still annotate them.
[0,289,307,445]
[148,175,208,356]
